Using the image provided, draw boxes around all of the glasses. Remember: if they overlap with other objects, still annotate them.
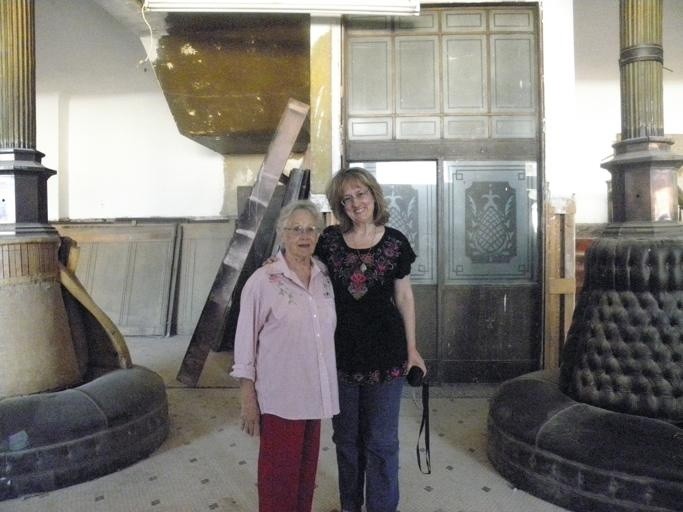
[283,225,318,235]
[340,187,370,206]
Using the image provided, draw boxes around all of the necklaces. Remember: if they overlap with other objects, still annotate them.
[350,224,377,274]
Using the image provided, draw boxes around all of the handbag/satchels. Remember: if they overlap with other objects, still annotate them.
[405,364,424,387]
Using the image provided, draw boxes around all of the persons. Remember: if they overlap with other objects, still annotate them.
[260,167,427,512]
[227,200,340,512]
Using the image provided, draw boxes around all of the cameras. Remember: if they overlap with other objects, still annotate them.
[406,366,424,388]
